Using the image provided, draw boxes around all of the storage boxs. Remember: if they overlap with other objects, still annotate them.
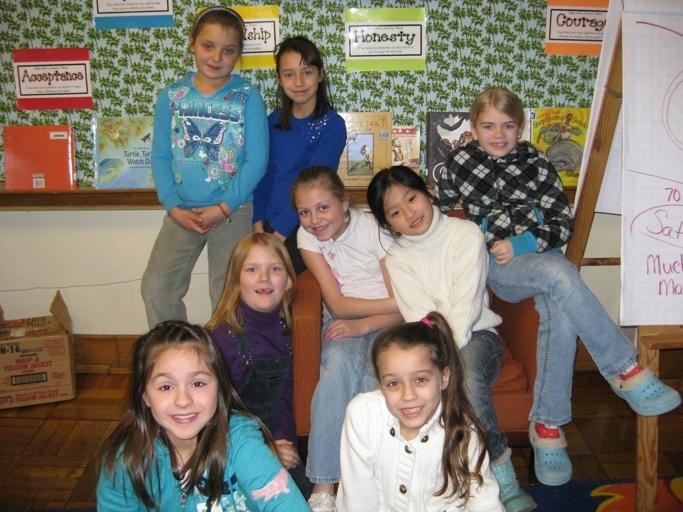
[0,288,77,413]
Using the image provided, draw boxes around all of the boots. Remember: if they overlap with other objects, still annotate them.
[490,448,537,512]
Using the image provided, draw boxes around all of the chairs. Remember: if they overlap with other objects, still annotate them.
[289,209,540,486]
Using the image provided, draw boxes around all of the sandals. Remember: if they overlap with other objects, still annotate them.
[529,418,573,487]
[607,368,682,417]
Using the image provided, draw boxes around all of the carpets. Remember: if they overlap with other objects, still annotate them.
[519,476,681,511]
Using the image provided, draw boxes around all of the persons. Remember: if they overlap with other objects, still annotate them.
[364,154,371,168]
[203,232,314,504]
[251,36,347,278]
[391,138,408,167]
[333,311,507,511]
[95,319,313,512]
[431,86,682,487]
[366,166,537,512]
[296,166,406,512]
[140,6,270,333]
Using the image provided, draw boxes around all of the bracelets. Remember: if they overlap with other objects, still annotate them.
[218,204,232,224]
